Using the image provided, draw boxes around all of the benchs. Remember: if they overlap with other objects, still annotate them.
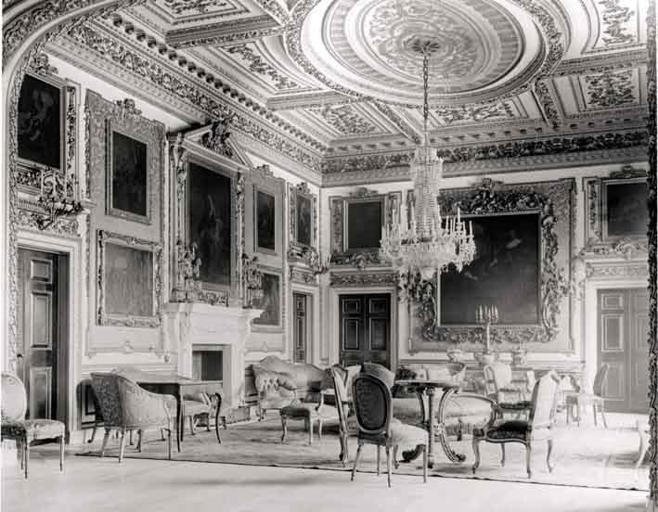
[251,355,335,421]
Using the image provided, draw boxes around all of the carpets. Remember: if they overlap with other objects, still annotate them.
[74,414,613,487]
[71,419,650,496]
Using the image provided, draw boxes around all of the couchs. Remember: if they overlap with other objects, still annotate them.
[483,362,536,419]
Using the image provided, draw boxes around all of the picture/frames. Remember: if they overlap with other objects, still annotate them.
[292,185,311,244]
[598,174,648,243]
[103,121,156,227]
[433,208,543,330]
[337,196,386,250]
[13,67,71,176]
[250,182,279,258]
[95,227,159,330]
[250,270,285,339]
[184,154,234,296]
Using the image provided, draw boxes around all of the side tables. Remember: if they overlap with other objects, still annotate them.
[281,400,324,446]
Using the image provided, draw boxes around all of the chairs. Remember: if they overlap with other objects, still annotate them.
[113,372,178,463]
[472,369,561,479]
[427,363,466,387]
[88,371,143,458]
[2,371,67,480]
[351,372,428,487]
[180,392,212,435]
[630,412,650,468]
[563,362,610,428]
[363,362,395,390]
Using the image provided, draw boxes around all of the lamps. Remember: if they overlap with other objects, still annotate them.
[377,39,480,286]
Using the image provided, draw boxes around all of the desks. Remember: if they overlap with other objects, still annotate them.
[136,380,224,442]
[395,379,466,470]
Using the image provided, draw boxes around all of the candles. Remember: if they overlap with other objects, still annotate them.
[472,301,499,326]
